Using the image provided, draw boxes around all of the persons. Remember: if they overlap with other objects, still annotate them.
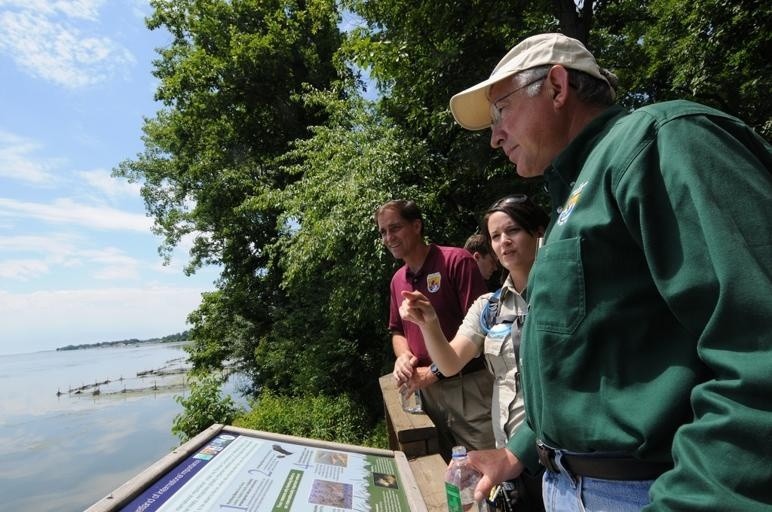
[376,197,495,452]
[463,234,499,281]
[448,32,770,512]
[398,192,550,511]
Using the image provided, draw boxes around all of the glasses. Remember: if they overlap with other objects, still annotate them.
[490,75,548,131]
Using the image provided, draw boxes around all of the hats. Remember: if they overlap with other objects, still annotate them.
[449,33,616,131]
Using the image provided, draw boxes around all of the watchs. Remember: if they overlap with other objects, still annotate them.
[431,363,444,382]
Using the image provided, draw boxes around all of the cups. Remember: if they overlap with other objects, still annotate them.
[401,387,422,412]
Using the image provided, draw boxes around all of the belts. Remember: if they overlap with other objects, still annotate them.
[444,356,486,379]
[535,439,671,480]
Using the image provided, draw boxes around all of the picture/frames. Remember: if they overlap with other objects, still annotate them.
[75,422,427,512]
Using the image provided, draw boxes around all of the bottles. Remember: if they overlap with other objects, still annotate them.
[446,445,486,512]
